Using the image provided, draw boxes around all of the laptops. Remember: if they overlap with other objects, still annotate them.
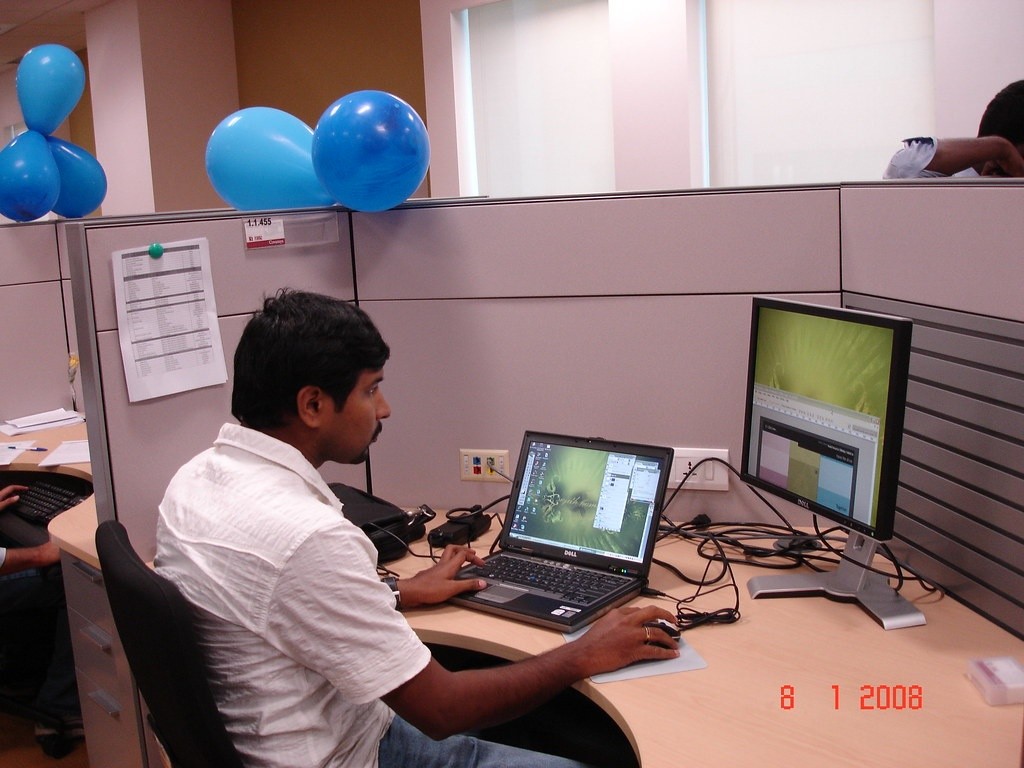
[444,429,674,634]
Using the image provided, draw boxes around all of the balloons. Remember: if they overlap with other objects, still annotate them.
[205,90,431,214]
[0,43,107,223]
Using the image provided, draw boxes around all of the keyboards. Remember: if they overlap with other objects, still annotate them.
[4,477,91,527]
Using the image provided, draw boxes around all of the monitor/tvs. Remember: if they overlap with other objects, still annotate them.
[740,297,927,631]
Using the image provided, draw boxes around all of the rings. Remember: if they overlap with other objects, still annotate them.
[644,626,651,642]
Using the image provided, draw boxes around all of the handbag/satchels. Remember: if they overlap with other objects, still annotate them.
[327,482,437,564]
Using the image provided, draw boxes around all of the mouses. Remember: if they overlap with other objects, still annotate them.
[643,622,681,650]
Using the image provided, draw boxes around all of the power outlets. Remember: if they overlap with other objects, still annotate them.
[667,447,730,492]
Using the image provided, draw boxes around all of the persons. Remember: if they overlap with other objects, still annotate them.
[0,485,60,689]
[882,79,1024,181]
[153,285,680,768]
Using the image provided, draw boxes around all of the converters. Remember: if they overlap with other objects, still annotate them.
[427,513,492,548]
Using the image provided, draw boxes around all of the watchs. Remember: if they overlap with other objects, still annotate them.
[381,576,401,609]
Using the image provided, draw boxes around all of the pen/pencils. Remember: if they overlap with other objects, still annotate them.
[8,446,48,451]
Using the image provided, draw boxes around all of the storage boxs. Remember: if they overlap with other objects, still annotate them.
[967,654,1024,706]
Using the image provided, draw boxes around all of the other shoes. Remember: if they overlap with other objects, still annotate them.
[35,710,84,737]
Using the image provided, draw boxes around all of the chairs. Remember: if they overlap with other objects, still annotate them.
[95,520,248,768]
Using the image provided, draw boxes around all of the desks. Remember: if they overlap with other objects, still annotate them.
[378,506,1024,768]
[0,411,149,768]
